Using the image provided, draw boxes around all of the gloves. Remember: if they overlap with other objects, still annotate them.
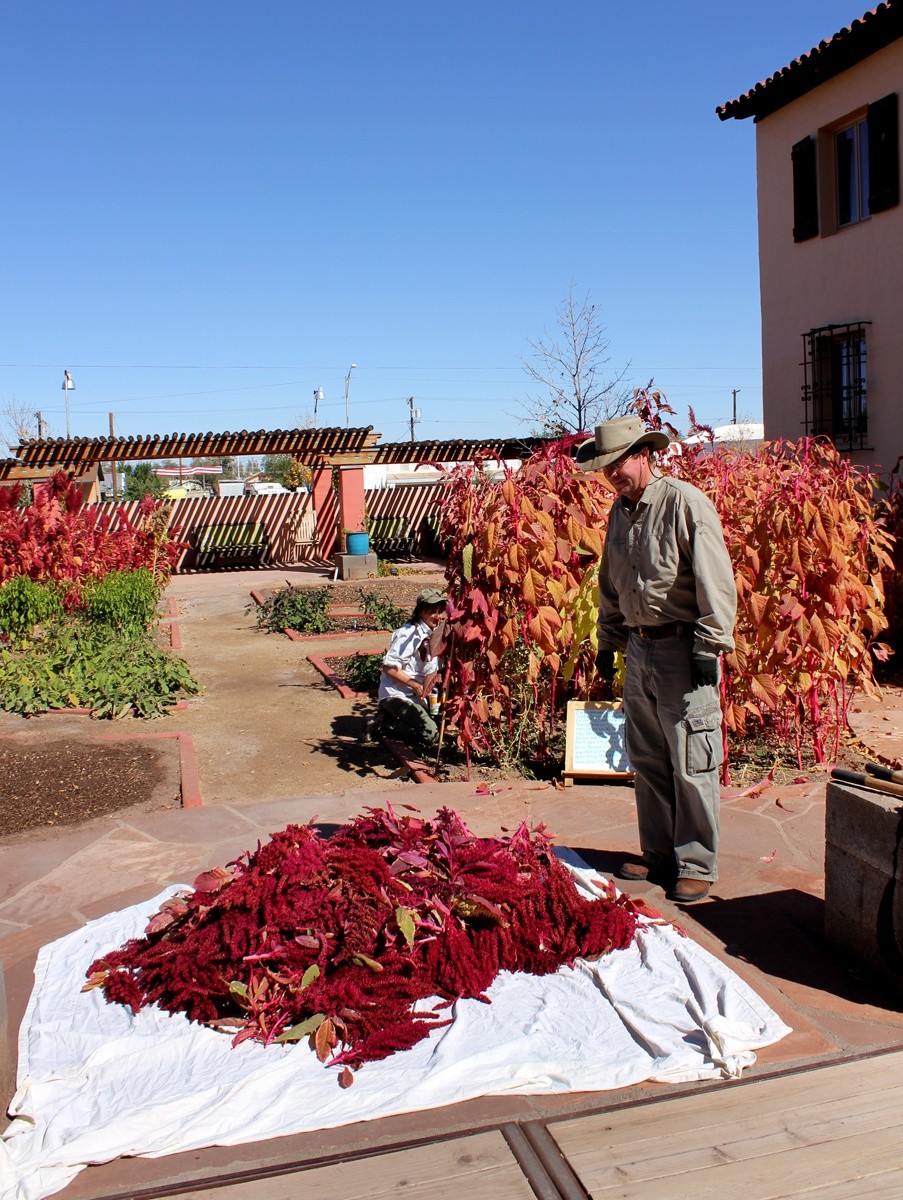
[690,653,718,690]
[595,650,618,681]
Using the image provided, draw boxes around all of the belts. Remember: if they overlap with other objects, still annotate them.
[631,623,677,641]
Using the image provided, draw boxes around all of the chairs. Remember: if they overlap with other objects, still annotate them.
[290,510,320,564]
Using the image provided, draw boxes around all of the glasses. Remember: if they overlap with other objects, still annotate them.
[598,454,634,473]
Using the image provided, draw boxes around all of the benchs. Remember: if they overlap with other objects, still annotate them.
[191,522,272,570]
[424,515,456,553]
[366,516,416,562]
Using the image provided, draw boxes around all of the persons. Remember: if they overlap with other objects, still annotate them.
[574,415,738,904]
[363,587,450,745]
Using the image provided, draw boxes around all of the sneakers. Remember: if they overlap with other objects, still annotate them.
[672,878,711,901]
[620,857,649,880]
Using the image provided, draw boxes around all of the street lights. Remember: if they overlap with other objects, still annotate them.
[60,368,77,439]
[311,386,325,427]
[343,362,358,428]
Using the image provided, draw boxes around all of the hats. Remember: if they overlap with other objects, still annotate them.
[576,416,670,471]
[416,587,448,605]
